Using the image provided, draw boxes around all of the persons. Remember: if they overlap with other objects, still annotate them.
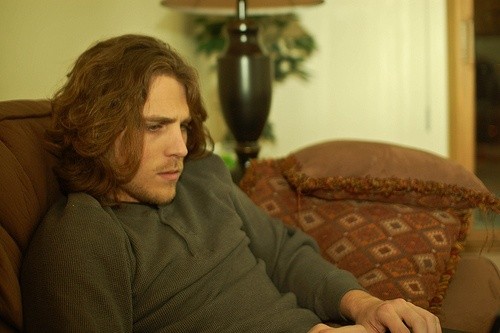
[23,32,441,333]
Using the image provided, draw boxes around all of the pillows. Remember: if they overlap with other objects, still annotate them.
[277,137,500,215]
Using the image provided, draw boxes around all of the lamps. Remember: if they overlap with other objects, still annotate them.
[160,0,322,166]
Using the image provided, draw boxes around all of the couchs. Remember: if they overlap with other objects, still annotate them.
[0,97,499,333]
